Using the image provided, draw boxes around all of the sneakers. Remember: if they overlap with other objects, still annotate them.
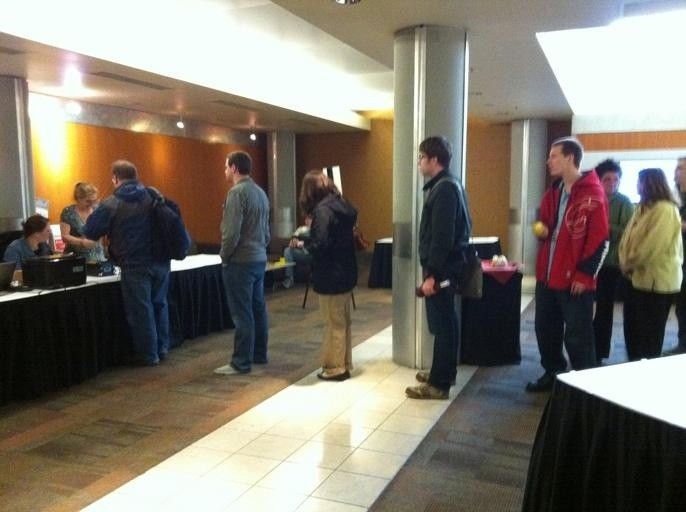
[406,372,456,398]
[213,364,243,374]
[283,277,294,288]
[116,352,165,366]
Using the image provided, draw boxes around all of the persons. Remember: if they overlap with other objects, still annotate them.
[2,214,54,270]
[587,156,686,370]
[58,181,105,258]
[84,160,172,366]
[297,168,358,382]
[351,225,375,261]
[404,133,474,400]
[282,214,314,288]
[526,136,609,390]
[212,148,273,378]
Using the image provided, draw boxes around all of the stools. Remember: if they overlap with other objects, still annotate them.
[303,277,355,311]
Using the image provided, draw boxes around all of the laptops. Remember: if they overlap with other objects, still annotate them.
[0,262,16,290]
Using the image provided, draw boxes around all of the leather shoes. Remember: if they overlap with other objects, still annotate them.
[317,369,350,380]
[527,371,555,391]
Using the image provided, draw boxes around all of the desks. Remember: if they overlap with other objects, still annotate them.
[522,353,686,511]
[367,235,501,288]
[0,253,235,409]
[264,261,297,298]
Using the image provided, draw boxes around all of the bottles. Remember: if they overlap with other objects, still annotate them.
[414,277,451,297]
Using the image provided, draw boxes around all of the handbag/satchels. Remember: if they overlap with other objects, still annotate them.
[353,225,369,251]
[455,255,483,298]
[147,187,191,260]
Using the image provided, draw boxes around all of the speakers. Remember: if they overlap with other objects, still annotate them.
[21,256,86,288]
